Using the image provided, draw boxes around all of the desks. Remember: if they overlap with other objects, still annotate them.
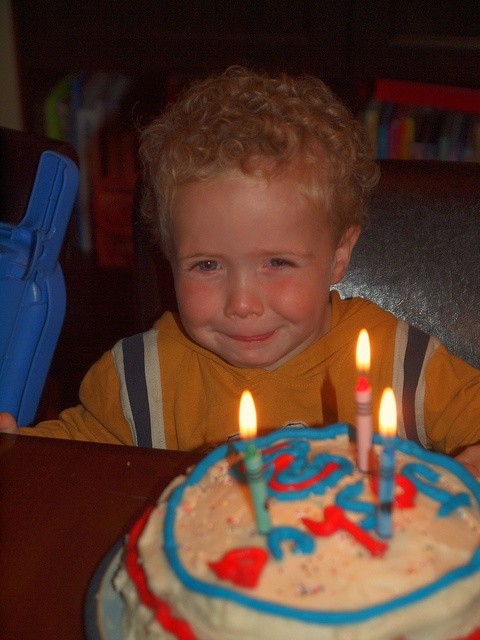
[1,430,215,640]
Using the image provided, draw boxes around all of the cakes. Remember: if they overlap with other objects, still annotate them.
[81,423,480,640]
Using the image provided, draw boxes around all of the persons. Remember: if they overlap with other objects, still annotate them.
[1,63,480,481]
